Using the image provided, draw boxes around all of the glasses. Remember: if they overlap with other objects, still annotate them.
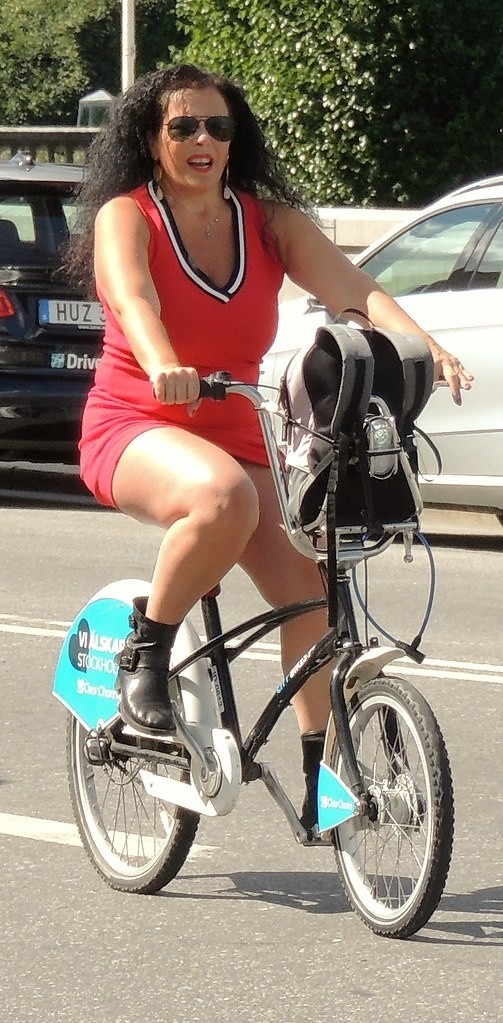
[161,116,236,142]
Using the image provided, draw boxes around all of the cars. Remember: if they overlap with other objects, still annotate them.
[1,152,106,465]
[259,177,503,532]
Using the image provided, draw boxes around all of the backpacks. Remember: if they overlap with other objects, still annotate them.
[275,308,442,550]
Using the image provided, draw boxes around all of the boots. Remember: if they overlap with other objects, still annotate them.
[298,731,327,842]
[114,596,183,736]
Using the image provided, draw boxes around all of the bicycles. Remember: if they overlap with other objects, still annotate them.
[51,369,468,940]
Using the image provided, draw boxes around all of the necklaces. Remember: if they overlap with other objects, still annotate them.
[173,195,225,239]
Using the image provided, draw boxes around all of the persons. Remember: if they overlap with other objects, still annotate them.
[77,64,473,842]
